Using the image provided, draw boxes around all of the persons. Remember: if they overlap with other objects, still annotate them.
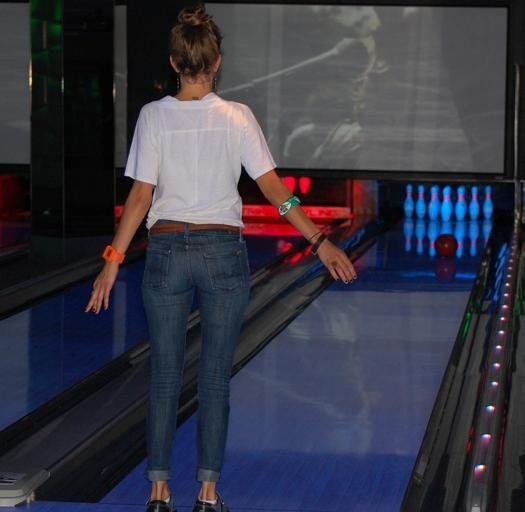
[82,5,359,511]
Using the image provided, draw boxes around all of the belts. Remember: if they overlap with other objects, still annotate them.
[149,224,239,235]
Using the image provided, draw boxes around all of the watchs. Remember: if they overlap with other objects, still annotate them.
[101,245,124,264]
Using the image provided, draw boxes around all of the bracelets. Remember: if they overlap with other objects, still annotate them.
[277,196,301,218]
[310,233,327,257]
[308,230,322,242]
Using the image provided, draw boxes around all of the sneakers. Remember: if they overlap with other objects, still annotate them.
[146,495,177,512]
[193,489,228,512]
